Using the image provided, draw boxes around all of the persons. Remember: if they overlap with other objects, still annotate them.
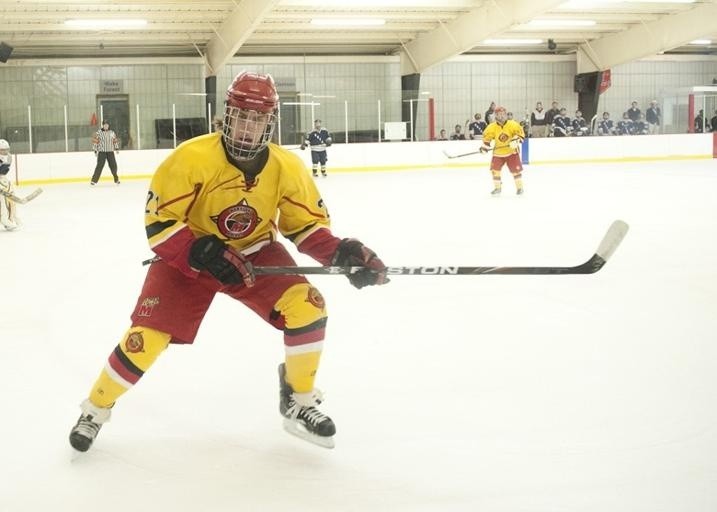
[301,120,332,176]
[480,106,526,195]
[91,119,121,185]
[711,111,717,131]
[694,110,710,133]
[70,70,390,452]
[0,139,17,230]
[438,101,660,141]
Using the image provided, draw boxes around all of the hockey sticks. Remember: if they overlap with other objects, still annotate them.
[0,188,43,205]
[251,221,628,276]
[442,145,509,158]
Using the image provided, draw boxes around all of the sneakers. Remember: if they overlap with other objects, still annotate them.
[280,383,336,436]
[516,181,524,194]
[313,170,319,176]
[70,398,111,451]
[321,170,327,176]
[491,183,502,194]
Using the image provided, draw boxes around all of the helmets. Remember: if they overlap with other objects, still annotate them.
[1,138,11,151]
[222,67,280,160]
[314,118,321,122]
[495,106,507,125]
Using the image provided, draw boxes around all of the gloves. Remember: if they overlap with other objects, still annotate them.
[187,234,255,288]
[301,143,308,150]
[326,139,332,146]
[328,238,391,289]
[510,139,519,149]
[479,143,490,154]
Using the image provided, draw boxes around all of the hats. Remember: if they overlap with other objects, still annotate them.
[102,120,110,124]
